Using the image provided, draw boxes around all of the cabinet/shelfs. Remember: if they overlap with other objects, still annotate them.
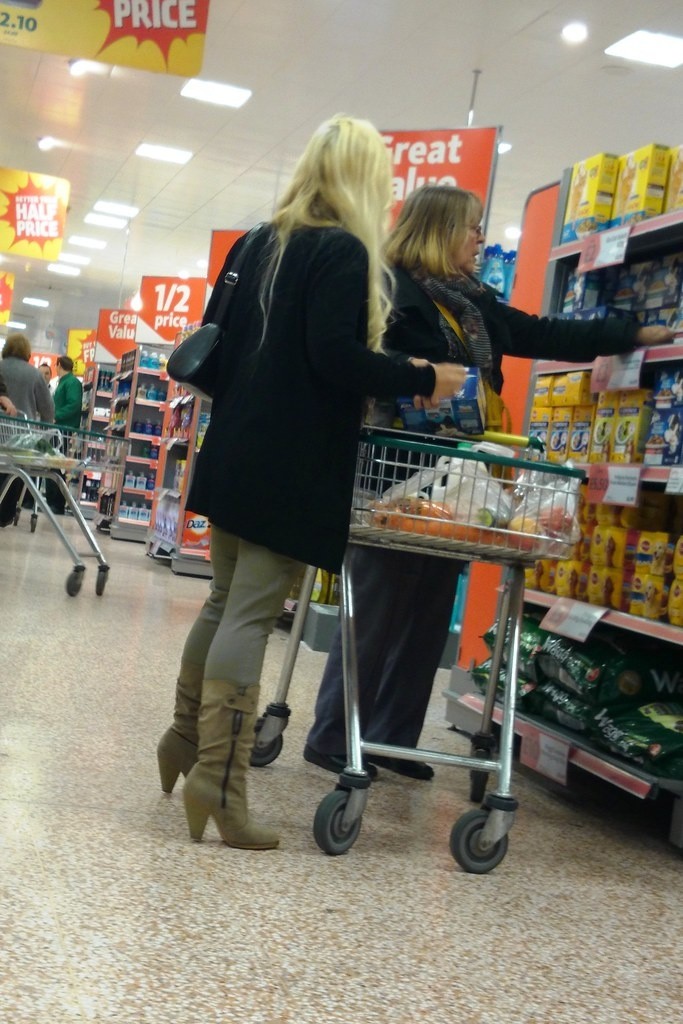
[77,333,213,576]
[446,165,683,801]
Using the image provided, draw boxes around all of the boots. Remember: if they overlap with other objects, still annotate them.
[157,657,204,793]
[182,680,279,850]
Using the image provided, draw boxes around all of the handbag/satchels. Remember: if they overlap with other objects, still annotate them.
[166,222,268,403]
[482,388,513,490]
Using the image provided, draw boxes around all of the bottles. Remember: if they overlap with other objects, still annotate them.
[71,456,92,477]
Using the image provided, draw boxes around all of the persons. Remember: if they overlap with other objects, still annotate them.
[301,186,674,781]
[157,114,469,850]
[0,333,83,528]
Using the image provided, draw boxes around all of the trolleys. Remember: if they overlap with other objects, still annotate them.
[0,401,137,597]
[255,423,593,874]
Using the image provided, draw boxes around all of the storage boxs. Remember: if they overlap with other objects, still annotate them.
[520,142,683,626]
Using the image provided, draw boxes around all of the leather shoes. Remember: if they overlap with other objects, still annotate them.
[365,753,435,779]
[303,745,377,779]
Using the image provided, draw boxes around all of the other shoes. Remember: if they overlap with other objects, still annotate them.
[37,504,65,514]
[0,518,13,527]
